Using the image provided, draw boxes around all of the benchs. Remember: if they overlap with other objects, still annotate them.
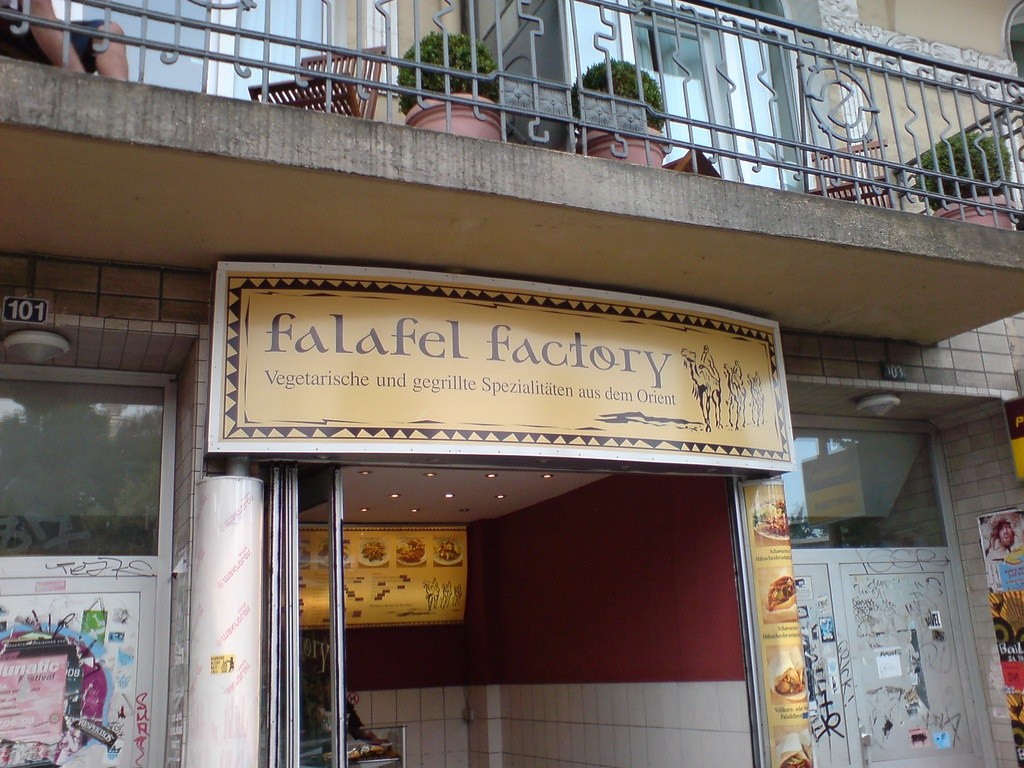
[806,139,892,208]
[249,46,387,121]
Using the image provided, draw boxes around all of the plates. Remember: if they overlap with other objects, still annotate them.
[754,529,790,541]
[433,539,464,565]
[771,685,806,700]
[396,539,426,565]
[358,540,389,566]
[319,557,351,567]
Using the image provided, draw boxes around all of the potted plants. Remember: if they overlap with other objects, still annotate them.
[395,29,502,142]
[910,134,1016,230]
[570,59,670,168]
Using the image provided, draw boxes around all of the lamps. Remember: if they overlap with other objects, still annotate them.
[2,330,71,366]
[855,395,901,418]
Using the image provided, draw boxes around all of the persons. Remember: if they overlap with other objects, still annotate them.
[980,511,1024,593]
[0,0,131,82]
[299,668,392,756]
